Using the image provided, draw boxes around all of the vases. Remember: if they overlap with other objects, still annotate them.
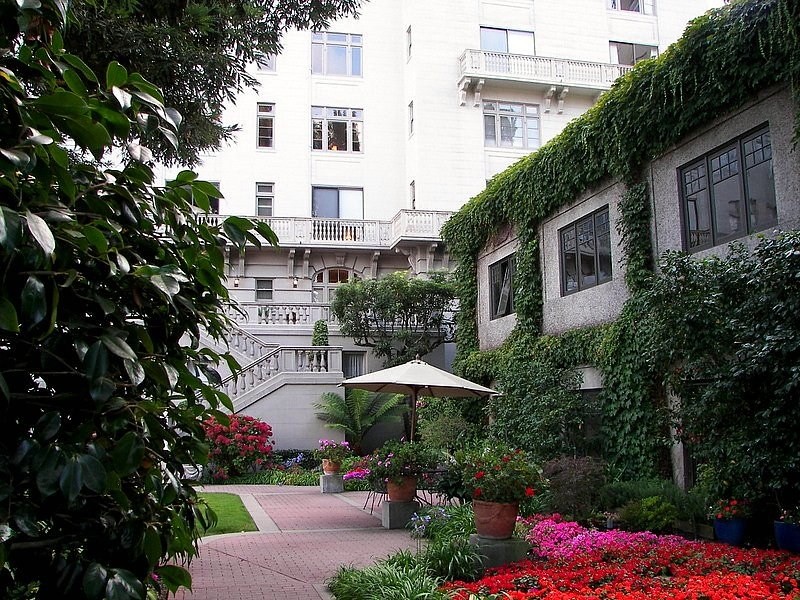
[472,499,519,539]
[388,477,416,502]
[714,517,744,546]
[322,459,341,475]
[773,521,800,555]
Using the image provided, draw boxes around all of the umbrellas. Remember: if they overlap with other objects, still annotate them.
[336,354,501,449]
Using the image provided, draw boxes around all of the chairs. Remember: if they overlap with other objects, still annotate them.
[364,475,389,515]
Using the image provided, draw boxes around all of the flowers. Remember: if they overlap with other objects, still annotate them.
[463,447,549,502]
[318,439,351,462]
[706,496,751,520]
[369,439,430,482]
[779,506,800,527]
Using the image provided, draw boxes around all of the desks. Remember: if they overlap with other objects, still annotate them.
[408,468,448,508]
